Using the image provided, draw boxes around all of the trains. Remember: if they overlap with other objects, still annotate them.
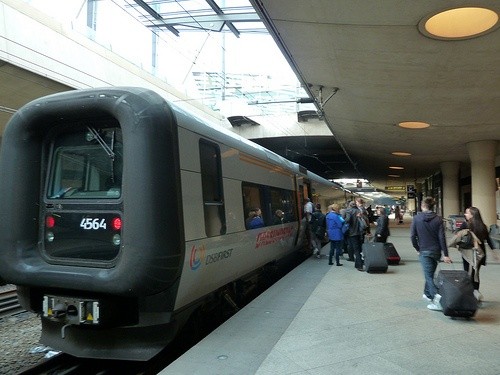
[0,86,374,361]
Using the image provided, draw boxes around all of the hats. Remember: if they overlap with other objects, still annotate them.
[349,202,356,206]
[357,199,363,204]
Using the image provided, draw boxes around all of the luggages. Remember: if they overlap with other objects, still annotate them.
[377,237,400,265]
[362,237,387,273]
[437,261,476,321]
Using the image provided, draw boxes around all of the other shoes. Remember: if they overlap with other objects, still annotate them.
[314,253,321,259]
[427,304,443,310]
[329,263,334,265]
[313,248,319,254]
[358,268,365,271]
[347,258,354,261]
[339,256,343,258]
[477,301,486,308]
[337,264,343,266]
[423,294,432,302]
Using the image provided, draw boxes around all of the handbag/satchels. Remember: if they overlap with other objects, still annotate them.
[459,231,472,249]
[316,226,325,240]
[358,216,368,232]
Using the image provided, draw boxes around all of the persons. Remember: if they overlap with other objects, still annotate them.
[303,197,315,222]
[384,205,389,217]
[324,198,370,270]
[372,207,391,244]
[458,206,495,300]
[408,197,452,306]
[393,206,400,225]
[246,208,283,230]
[308,203,326,259]
[367,205,372,213]
